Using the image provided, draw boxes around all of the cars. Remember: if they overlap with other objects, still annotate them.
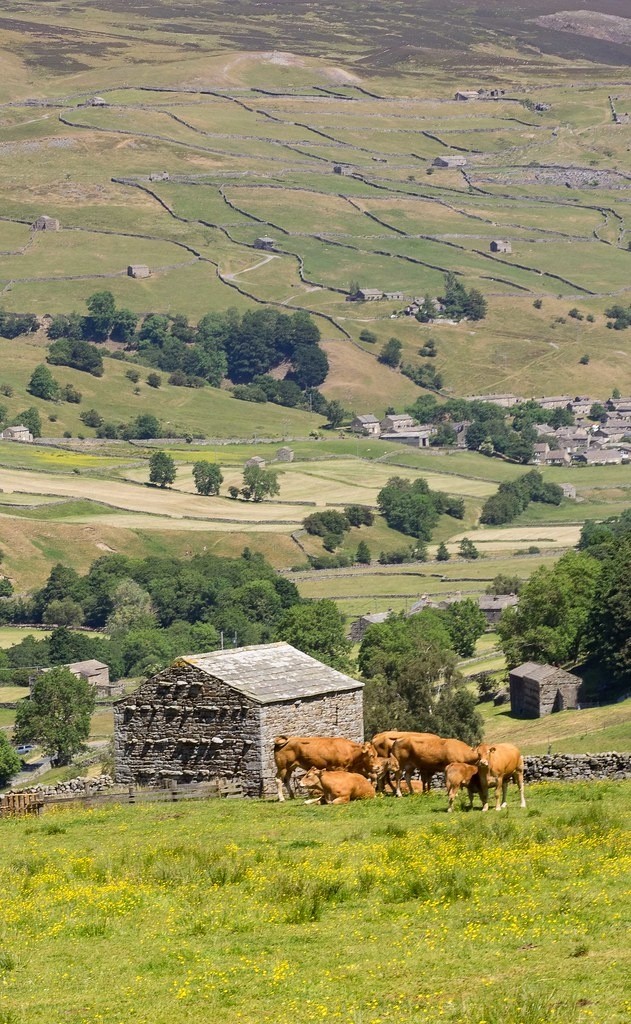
[17,745,33,755]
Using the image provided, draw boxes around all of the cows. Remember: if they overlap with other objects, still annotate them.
[274,730,527,813]
